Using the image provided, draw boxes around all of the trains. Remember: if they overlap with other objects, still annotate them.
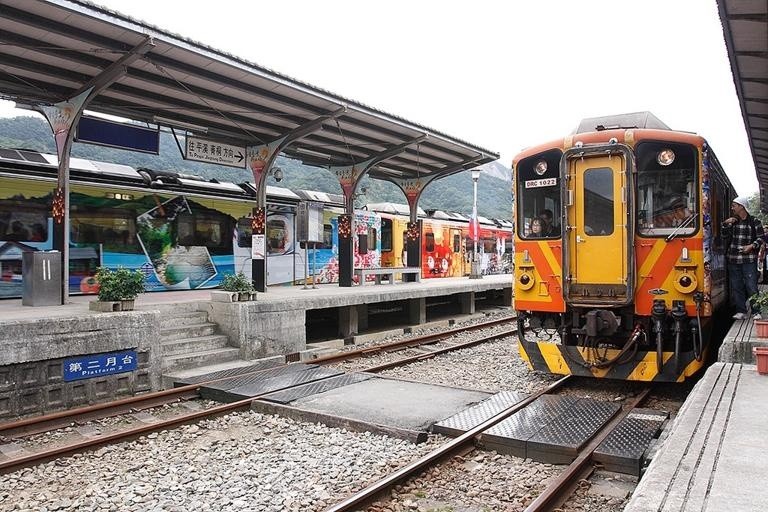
[509,111,739,383]
[0,148,513,298]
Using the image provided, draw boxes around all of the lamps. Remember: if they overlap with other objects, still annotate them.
[269,166,283,182]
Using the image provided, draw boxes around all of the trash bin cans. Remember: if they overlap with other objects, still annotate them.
[22,250,61,307]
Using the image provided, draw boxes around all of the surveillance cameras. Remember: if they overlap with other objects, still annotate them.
[273,171,284,182]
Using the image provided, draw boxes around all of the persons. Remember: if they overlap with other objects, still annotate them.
[526,217,556,237]
[4,221,29,241]
[720,197,765,320]
[757,223,768,284]
[539,209,560,236]
[27,223,46,242]
[666,198,693,228]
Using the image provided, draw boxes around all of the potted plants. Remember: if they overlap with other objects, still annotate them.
[220,272,256,302]
[751,290,768,338]
[97,266,146,311]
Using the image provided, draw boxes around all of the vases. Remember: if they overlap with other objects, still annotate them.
[752,346,768,374]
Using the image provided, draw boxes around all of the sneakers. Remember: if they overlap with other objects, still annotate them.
[733,313,747,319]
[758,281,768,285]
[753,314,762,320]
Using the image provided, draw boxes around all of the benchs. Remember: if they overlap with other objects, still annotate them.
[354,268,421,285]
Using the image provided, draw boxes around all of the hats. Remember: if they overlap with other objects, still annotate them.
[733,196,751,215]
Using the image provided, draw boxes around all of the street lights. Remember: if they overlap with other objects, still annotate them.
[467,169,484,279]
